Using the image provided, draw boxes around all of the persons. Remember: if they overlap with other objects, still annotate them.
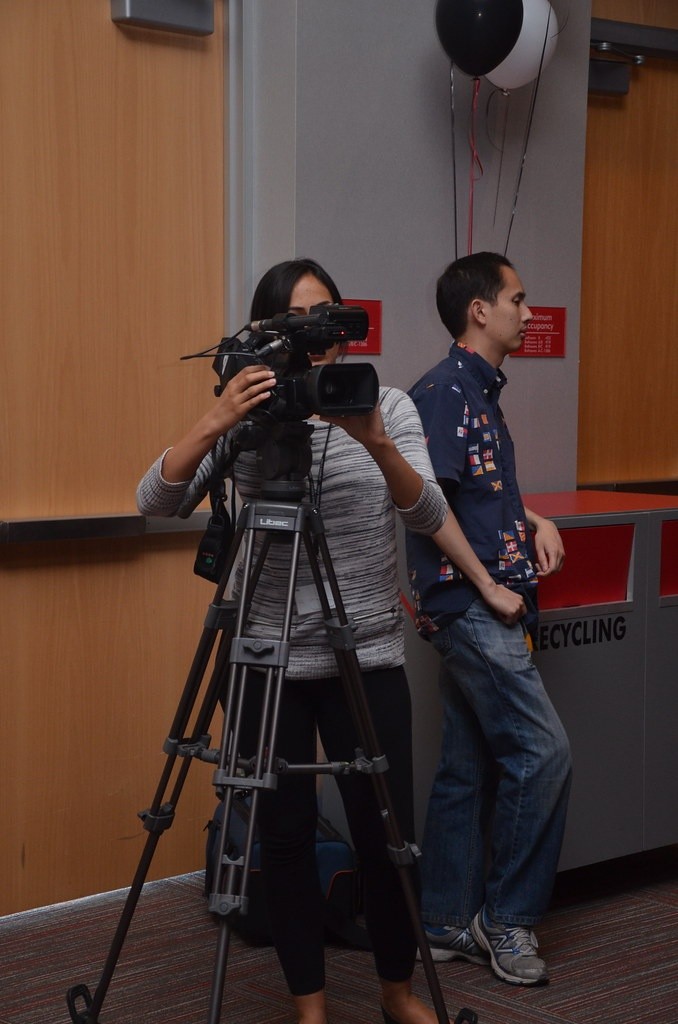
[133,258,482,1024]
[396,252,574,987]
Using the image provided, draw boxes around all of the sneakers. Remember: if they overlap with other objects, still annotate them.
[471,905,549,986]
[414,918,490,966]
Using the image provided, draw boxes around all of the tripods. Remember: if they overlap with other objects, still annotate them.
[85,422,451,1024]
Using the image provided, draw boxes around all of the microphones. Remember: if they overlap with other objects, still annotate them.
[244,312,328,333]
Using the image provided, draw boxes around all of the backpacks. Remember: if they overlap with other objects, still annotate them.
[205,791,360,946]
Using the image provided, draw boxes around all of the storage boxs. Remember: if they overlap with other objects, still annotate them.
[202,789,356,949]
